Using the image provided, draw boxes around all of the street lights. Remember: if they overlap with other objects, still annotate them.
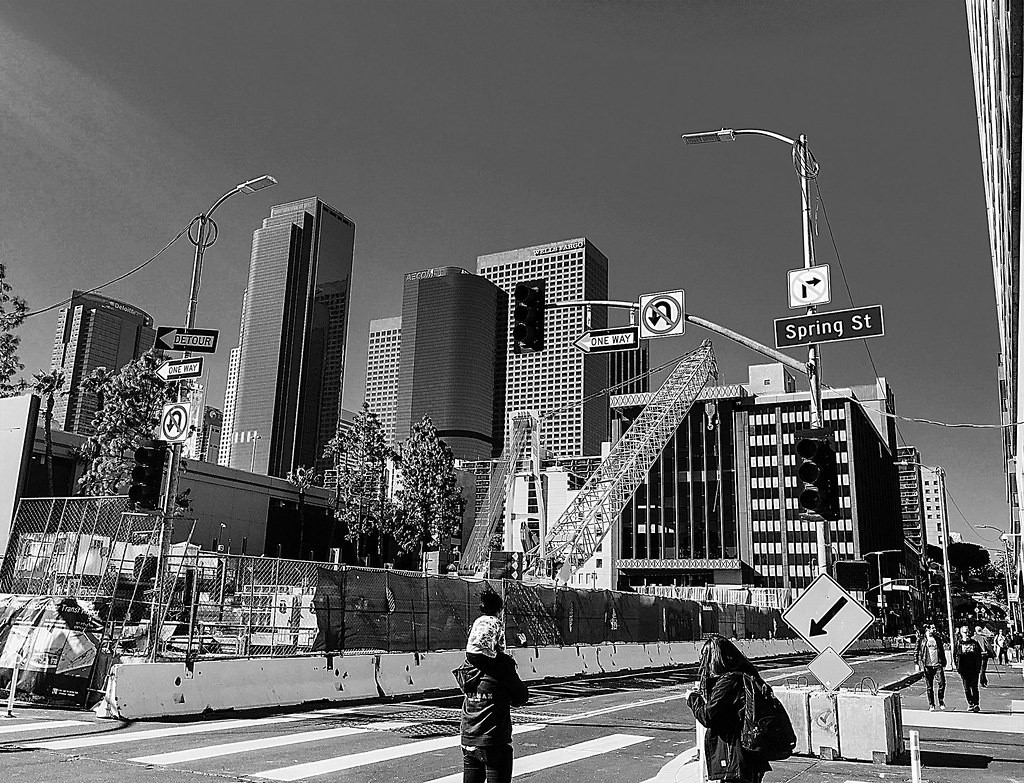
[862,547,900,635]
[810,556,818,580]
[975,523,1019,645]
[145,177,280,660]
[893,458,958,671]
[217,521,227,548]
[681,126,824,426]
[822,542,838,582]
[249,434,262,472]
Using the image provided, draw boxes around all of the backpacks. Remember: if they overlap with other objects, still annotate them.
[718,671,796,761]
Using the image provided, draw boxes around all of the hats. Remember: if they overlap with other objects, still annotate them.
[975,626,982,635]
[481,589,503,610]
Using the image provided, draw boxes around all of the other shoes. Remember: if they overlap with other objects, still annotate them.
[985,679,987,685]
[929,703,935,711]
[999,664,1002,665]
[939,701,946,710]
[980,684,986,687]
[974,705,980,712]
[967,705,974,713]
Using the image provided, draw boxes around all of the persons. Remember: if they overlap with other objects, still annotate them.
[953,625,983,713]
[887,624,1024,665]
[466,593,516,677]
[972,626,995,688]
[913,621,948,711]
[459,657,529,783]
[685,634,773,783]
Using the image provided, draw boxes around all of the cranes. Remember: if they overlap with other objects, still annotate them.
[453,336,720,585]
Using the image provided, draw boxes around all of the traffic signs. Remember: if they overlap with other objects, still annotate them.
[154,358,203,381]
[152,325,219,353]
[576,326,640,354]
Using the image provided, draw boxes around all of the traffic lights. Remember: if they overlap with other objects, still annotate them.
[513,276,544,354]
[792,426,840,521]
[128,440,167,508]
